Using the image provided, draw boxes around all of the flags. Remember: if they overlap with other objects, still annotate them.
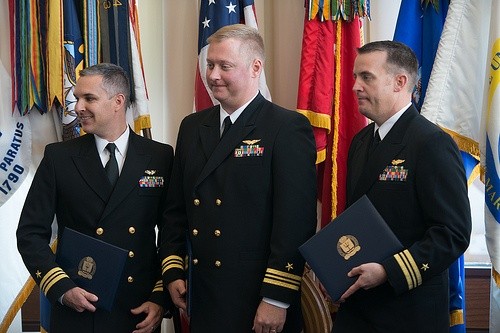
[297,0,371,227]
[193,0,271,114]
[393,0,500,333]
[10,0,150,142]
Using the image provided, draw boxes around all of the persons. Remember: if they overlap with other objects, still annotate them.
[330,42,472,333]
[16,62,175,333]
[154,24,317,333]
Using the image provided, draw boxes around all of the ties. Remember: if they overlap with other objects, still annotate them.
[105,143,119,186]
[221,116,232,139]
[374,128,381,148]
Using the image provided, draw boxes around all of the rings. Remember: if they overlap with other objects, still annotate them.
[270,329,276,333]
